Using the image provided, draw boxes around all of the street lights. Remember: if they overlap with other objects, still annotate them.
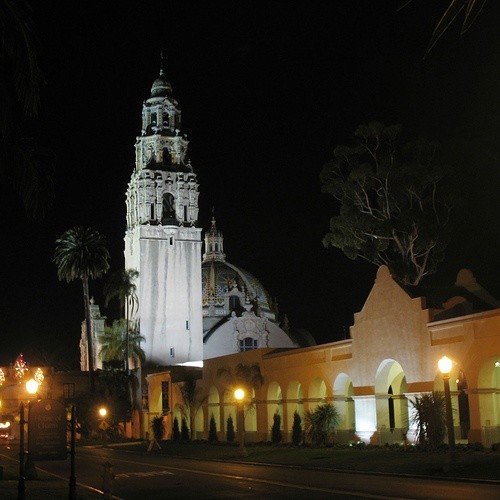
[234,389,248,457]
[99,408,108,447]
[438,355,458,459]
